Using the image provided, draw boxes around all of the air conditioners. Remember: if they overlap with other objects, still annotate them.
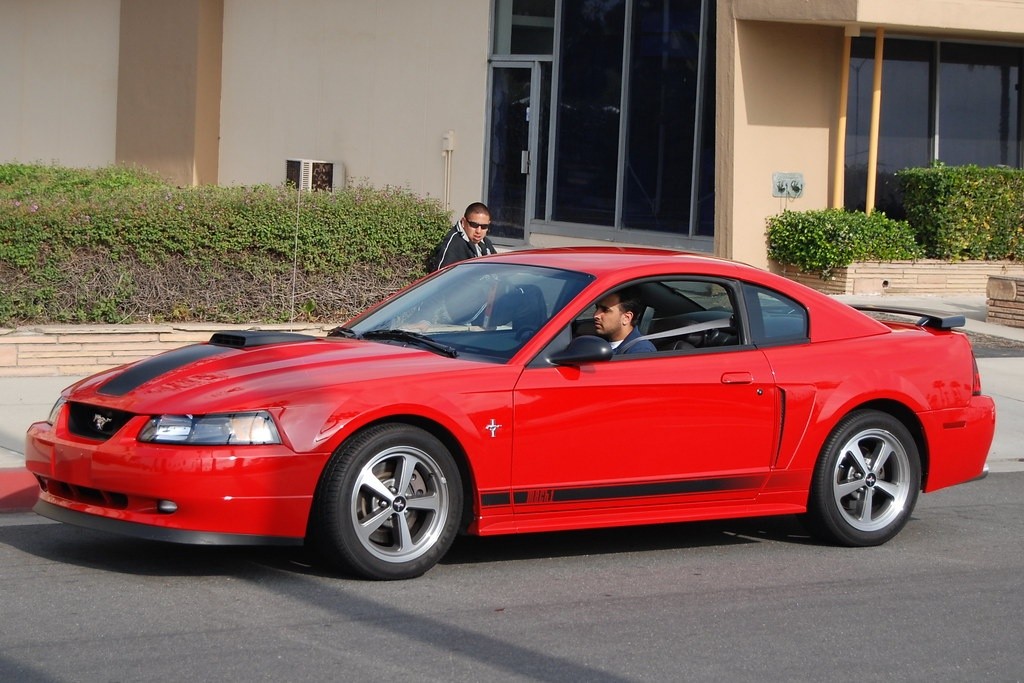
[284,158,345,195]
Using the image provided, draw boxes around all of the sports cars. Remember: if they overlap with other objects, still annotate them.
[22,248,998,585]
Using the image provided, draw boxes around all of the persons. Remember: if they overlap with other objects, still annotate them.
[593,287,657,355]
[398,202,548,334]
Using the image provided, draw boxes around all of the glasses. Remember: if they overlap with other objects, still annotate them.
[466,218,490,230]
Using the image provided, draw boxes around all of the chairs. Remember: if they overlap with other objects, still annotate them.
[511,284,548,333]
[667,322,734,352]
